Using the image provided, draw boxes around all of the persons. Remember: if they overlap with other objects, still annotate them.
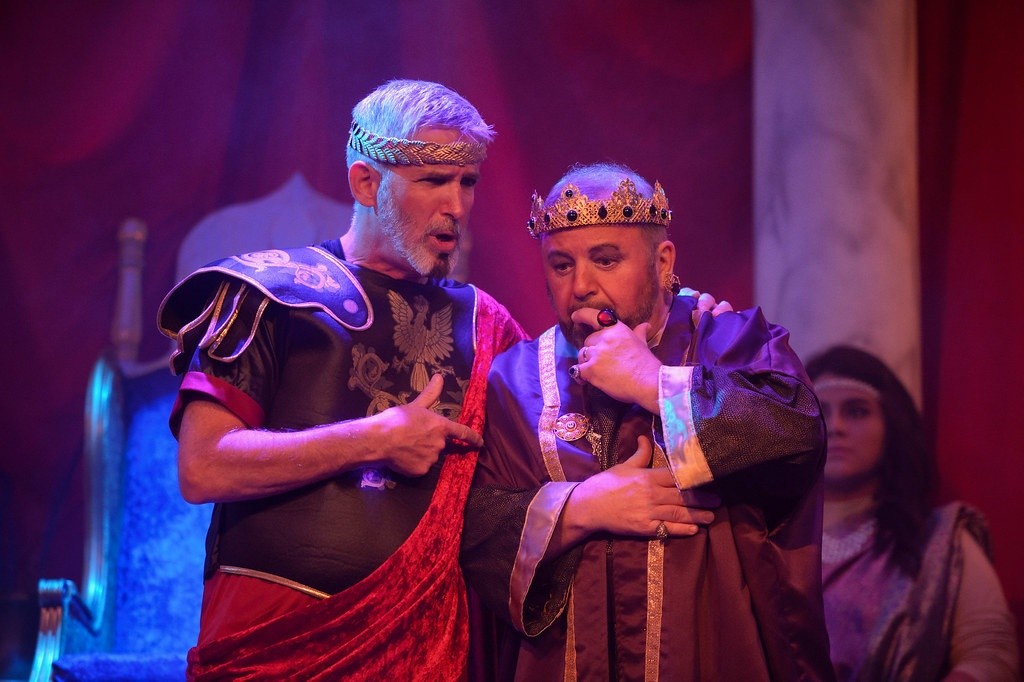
[799,339,1019,681]
[159,76,534,681]
[456,162,839,681]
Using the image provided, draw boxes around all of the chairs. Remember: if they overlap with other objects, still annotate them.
[24,328,208,682]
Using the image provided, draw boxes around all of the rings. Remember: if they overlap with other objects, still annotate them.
[655,519,668,543]
[568,365,587,385]
[597,309,617,328]
[583,345,591,363]
